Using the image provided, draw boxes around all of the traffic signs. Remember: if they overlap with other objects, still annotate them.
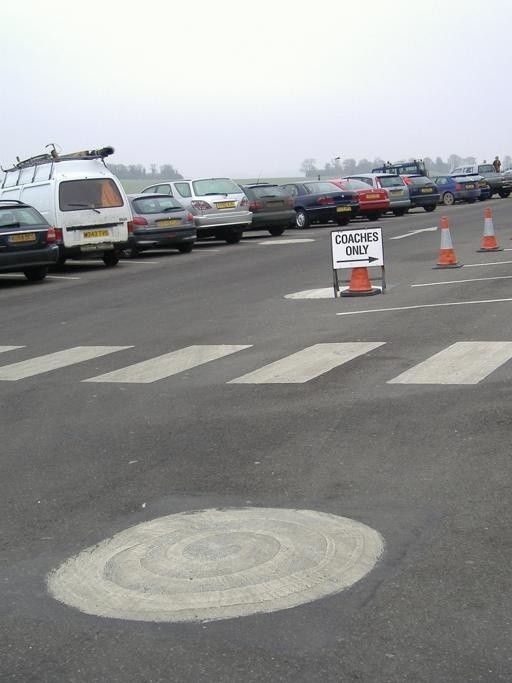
[329,229,384,267]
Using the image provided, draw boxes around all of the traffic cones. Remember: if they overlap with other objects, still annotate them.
[342,267,380,298]
[433,215,467,269]
[475,207,504,252]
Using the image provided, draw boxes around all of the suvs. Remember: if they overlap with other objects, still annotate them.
[430,172,481,205]
[364,159,441,212]
[140,176,253,247]
[0,200,59,284]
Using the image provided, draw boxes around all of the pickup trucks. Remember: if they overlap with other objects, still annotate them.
[449,163,512,200]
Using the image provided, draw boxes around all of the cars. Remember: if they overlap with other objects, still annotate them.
[119,193,197,258]
[287,179,359,229]
[240,183,297,239]
[460,171,491,202]
[330,179,390,223]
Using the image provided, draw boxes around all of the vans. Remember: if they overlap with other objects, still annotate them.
[0,146,135,268]
[339,173,411,217]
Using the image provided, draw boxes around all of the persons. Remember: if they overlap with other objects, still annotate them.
[483,159,486,163]
[493,155,500,171]
